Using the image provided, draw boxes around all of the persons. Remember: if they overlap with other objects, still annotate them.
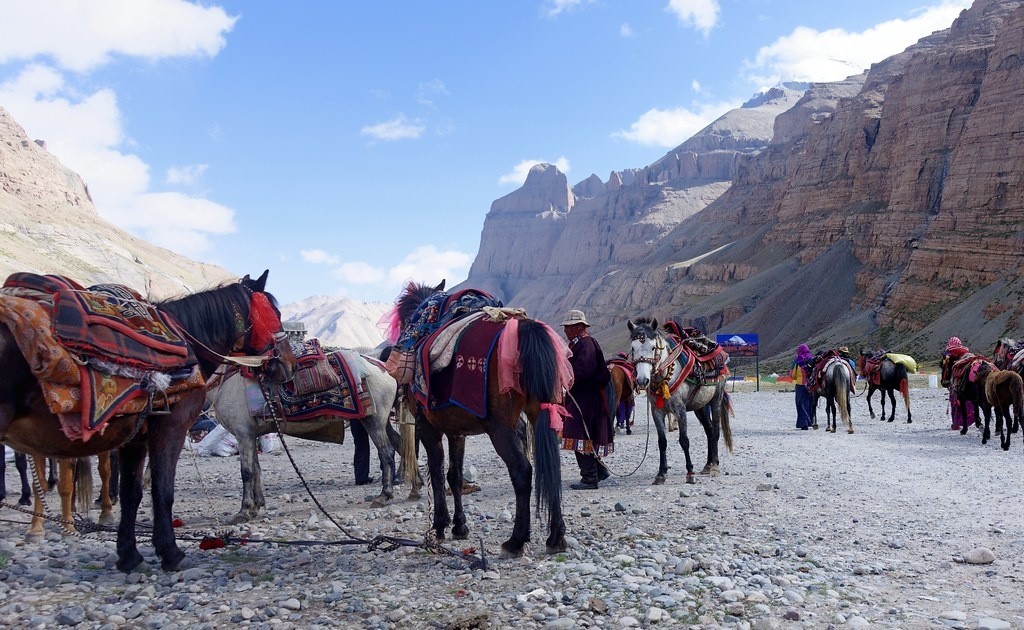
[557,310,615,490]
[349,416,397,484]
[189,398,217,442]
[939,337,976,430]
[617,351,634,427]
[839,346,856,372]
[790,344,816,430]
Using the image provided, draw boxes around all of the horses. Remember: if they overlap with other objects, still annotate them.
[603,316,739,486]
[0,269,569,576]
[808,349,857,434]
[859,348,913,424]
[938,335,1024,452]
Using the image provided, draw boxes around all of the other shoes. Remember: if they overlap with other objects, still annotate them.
[570,481,598,489]
[801,426,808,430]
[951,424,959,430]
[597,470,610,482]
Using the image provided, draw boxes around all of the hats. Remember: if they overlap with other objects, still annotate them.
[559,309,590,327]
[838,346,849,353]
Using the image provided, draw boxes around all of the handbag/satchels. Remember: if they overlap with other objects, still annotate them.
[385,344,415,385]
[212,432,240,457]
[259,433,273,452]
[199,426,229,450]
